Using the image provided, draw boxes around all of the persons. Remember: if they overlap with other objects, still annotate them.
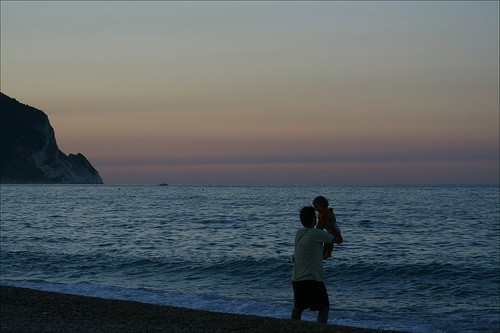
[289,205,338,323]
[311,195,344,258]
